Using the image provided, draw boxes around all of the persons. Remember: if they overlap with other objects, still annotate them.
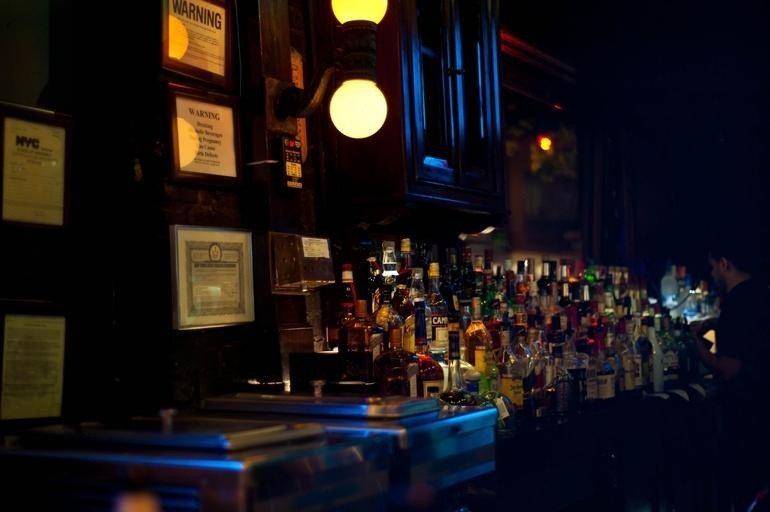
[686,236,770,511]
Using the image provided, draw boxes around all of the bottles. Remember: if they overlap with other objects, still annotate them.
[342,233,689,430]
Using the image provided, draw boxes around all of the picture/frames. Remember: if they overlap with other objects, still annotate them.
[0,303,65,426]
[170,224,255,330]
[168,82,243,183]
[0,101,71,234]
[161,0,231,88]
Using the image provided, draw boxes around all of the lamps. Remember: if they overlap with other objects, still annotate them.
[329,0,389,139]
